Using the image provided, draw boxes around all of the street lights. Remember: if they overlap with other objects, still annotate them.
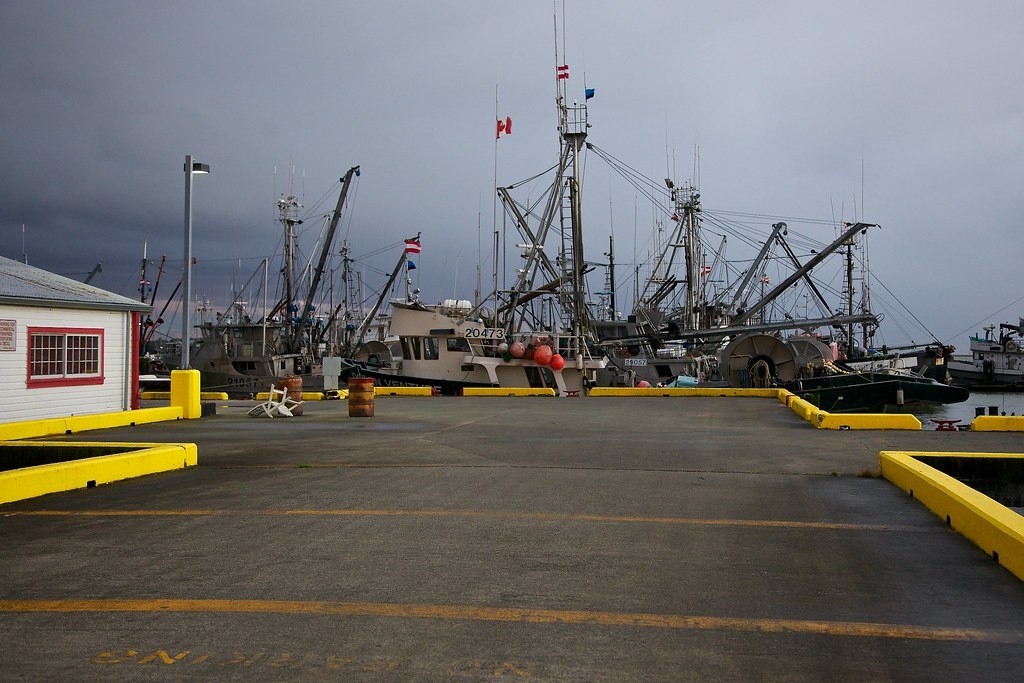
[176,155,210,371]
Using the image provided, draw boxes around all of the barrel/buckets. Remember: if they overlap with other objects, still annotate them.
[277,377,304,416]
[348,377,375,417]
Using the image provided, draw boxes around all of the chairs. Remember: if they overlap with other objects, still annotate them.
[246,384,305,419]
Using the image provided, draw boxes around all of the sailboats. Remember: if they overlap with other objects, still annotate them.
[137,1,956,397]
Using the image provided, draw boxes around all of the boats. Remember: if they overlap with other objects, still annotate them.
[945,316,1024,384]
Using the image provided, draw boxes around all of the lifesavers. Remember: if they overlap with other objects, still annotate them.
[535,336,554,349]
[368,354,380,364]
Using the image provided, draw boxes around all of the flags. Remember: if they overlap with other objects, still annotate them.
[586,88,595,100]
[496,117,512,139]
[404,239,422,253]
[408,261,416,270]
[557,64,569,80]
[671,213,679,222]
[701,266,711,277]
